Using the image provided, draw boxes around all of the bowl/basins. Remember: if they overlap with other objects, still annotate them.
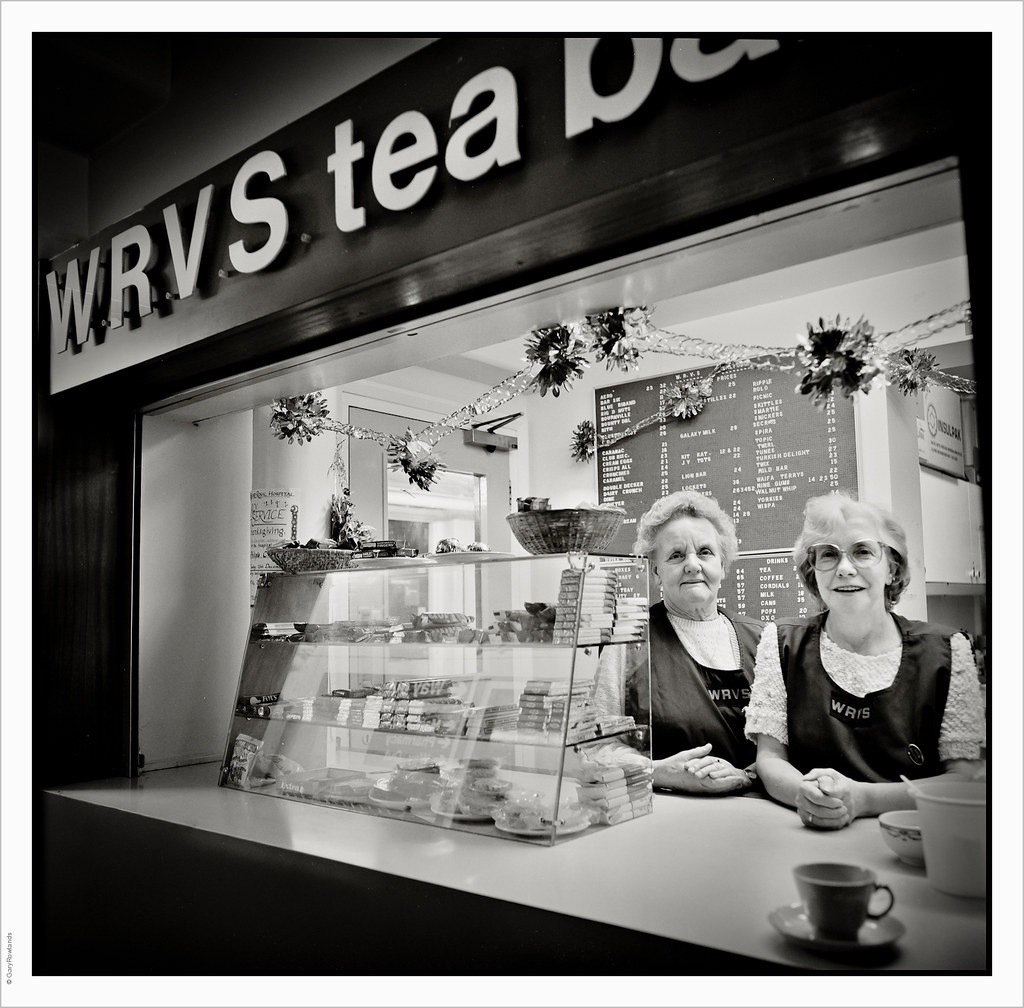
[878,809,927,867]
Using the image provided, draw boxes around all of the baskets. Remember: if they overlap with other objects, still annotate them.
[266,548,353,572]
[505,506,628,555]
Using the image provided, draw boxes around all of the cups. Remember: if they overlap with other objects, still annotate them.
[793,862,894,941]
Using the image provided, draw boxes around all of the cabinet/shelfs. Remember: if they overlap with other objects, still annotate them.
[218,552,654,847]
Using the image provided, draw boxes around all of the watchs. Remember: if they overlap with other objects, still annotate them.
[746,768,758,784]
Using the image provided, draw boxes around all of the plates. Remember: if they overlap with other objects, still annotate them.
[495,821,593,838]
[322,792,367,807]
[429,801,496,822]
[368,789,414,810]
[768,902,905,956]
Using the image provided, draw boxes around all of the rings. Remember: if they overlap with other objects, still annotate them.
[809,814,812,822]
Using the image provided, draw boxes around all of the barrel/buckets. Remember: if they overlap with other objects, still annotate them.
[906,782,986,899]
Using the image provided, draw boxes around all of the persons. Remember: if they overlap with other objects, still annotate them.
[577,492,769,796]
[742,492,983,829]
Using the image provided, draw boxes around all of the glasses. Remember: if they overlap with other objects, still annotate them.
[805,539,888,572]
